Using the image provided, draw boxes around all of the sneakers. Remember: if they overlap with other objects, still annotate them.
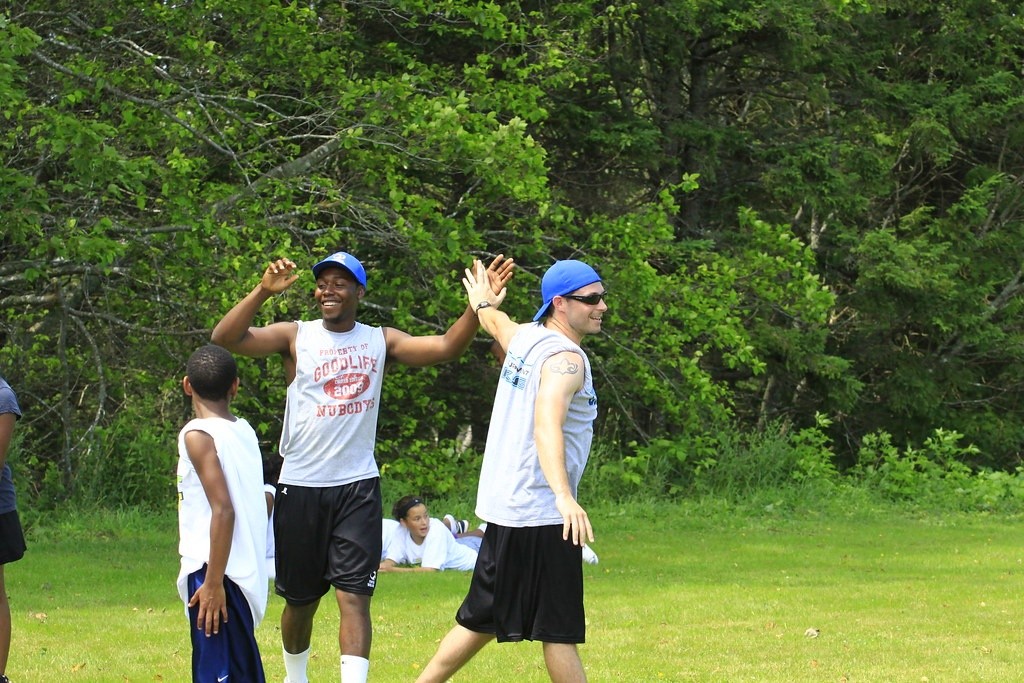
[456,520,468,535]
[581,543,598,565]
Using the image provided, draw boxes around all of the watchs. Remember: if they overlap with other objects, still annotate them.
[475,301,491,315]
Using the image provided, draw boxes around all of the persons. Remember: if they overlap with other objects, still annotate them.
[0,377,26,683]
[382,494,600,574]
[417,259,612,681]
[210,250,516,683]
[380,513,488,572]
[174,343,270,683]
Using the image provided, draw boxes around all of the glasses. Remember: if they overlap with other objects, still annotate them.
[561,292,608,305]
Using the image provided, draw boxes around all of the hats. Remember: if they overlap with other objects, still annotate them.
[312,251,366,289]
[533,258,601,321]
